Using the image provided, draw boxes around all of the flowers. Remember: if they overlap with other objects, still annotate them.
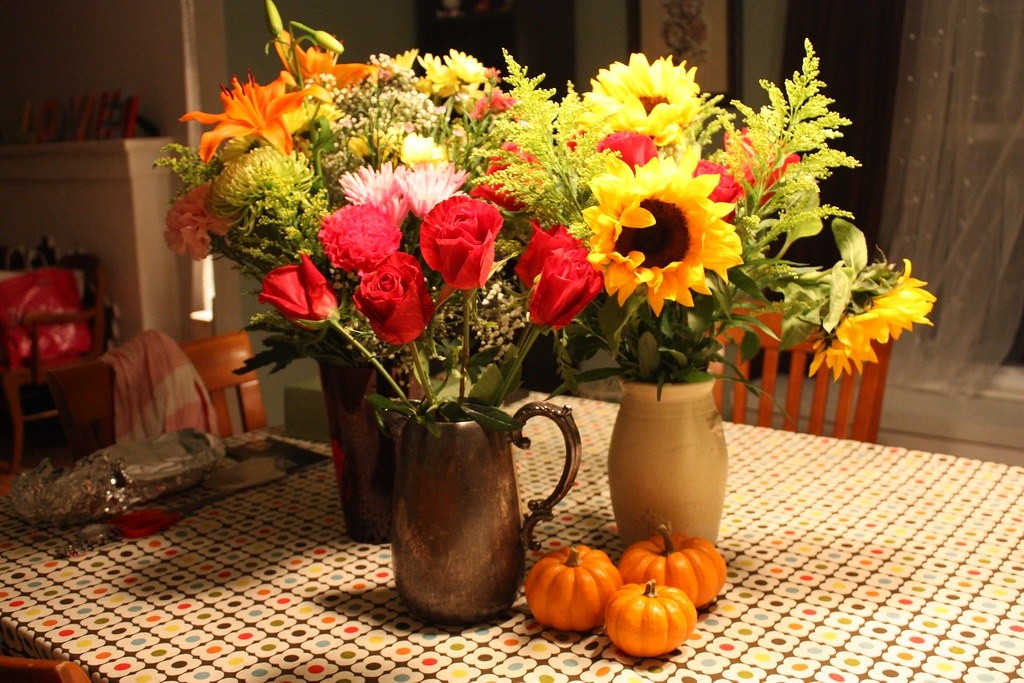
[465,38,942,386]
[149,0,474,359]
[260,134,609,430]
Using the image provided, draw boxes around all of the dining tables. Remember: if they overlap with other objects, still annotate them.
[1,384,1024,680]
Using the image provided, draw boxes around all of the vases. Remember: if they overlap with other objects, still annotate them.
[604,377,731,555]
[312,354,417,547]
[374,393,583,625]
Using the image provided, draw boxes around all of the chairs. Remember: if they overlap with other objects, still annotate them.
[49,327,277,469]
[703,297,894,446]
[1,250,115,475]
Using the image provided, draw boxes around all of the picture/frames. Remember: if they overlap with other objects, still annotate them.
[622,0,747,112]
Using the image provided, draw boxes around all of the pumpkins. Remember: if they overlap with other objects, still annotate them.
[526,525,728,657]
